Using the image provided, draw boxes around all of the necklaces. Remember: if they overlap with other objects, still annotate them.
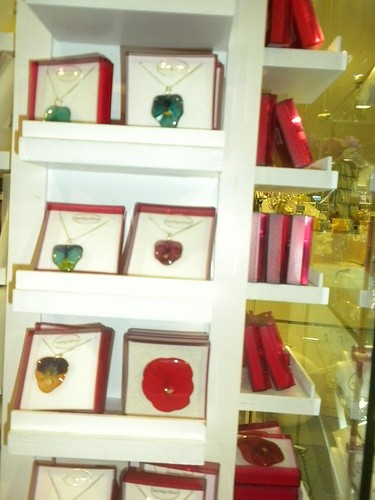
[136,485,193,499]
[153,466,207,479]
[148,215,203,265]
[52,213,109,271]
[47,472,104,500]
[45,66,95,122]
[36,338,90,393]
[141,63,201,127]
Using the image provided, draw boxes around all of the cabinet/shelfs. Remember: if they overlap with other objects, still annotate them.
[0,0,348,500]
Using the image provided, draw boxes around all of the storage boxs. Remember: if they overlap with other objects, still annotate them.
[244,311,296,394]
[16,321,210,419]
[234,421,301,500]
[267,0,325,49]
[256,88,314,169]
[26,460,220,500]
[248,211,316,285]
[26,46,223,131]
[28,202,216,281]
